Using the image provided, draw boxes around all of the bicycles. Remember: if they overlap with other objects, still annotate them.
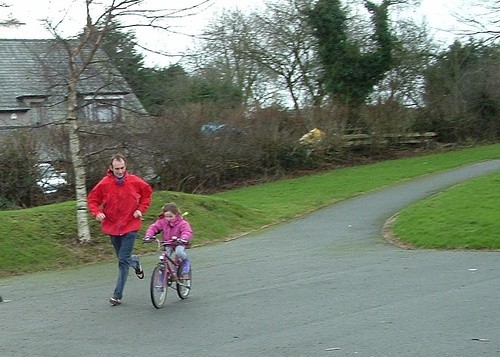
[142,236,193,309]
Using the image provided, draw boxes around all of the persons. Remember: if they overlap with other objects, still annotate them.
[145,203,193,291]
[88,152,152,304]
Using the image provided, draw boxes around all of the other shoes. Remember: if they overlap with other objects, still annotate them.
[179,274,190,281]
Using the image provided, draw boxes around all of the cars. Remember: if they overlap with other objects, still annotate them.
[33,163,67,196]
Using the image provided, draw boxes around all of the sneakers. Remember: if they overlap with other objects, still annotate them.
[136,261,144,279]
[110,297,121,305]
